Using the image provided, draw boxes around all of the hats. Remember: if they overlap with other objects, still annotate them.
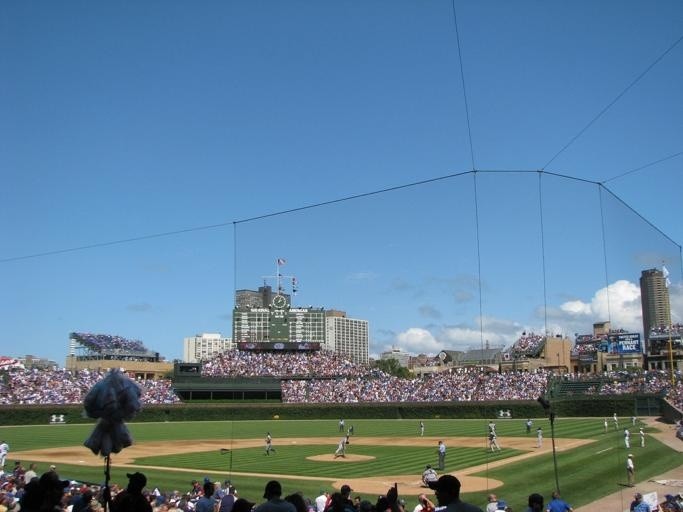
[263,480,281,499]
[126,472,147,487]
[428,475,461,490]
[38,472,70,488]
[204,477,209,482]
[496,500,506,508]
[341,485,353,493]
[224,480,230,484]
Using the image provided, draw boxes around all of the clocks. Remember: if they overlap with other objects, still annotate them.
[272,295,286,308]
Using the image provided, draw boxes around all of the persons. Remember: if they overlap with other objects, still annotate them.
[69,332,156,362]
[265,432,276,457]
[339,419,345,432]
[630,492,682,512]
[536,427,543,448]
[420,420,425,437]
[563,321,682,448]
[1,440,9,467]
[189,348,556,404]
[335,424,354,457]
[437,441,445,470]
[488,421,501,452]
[0,366,181,404]
[510,331,547,361]
[626,453,636,487]
[1,462,573,512]
[526,418,534,433]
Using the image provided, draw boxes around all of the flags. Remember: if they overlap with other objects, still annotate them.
[664,279,671,288]
[661,265,669,278]
[277,258,287,266]
